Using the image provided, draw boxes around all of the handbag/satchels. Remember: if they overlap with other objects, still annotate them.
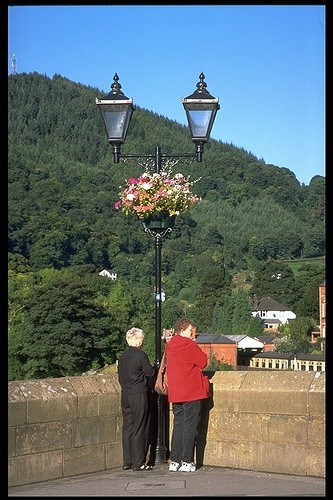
[154,350,168,395]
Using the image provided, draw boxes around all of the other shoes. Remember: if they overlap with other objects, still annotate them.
[123,464,152,471]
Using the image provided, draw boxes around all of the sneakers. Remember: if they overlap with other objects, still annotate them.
[169,462,196,472]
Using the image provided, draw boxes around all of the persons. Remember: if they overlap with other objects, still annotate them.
[165,318,208,472]
[117,327,154,471]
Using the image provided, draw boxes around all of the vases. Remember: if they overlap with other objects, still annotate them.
[138,210,177,230]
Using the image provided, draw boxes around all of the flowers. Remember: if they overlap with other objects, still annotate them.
[113,168,198,219]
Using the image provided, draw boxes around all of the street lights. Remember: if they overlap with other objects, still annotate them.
[95,71,222,472]
